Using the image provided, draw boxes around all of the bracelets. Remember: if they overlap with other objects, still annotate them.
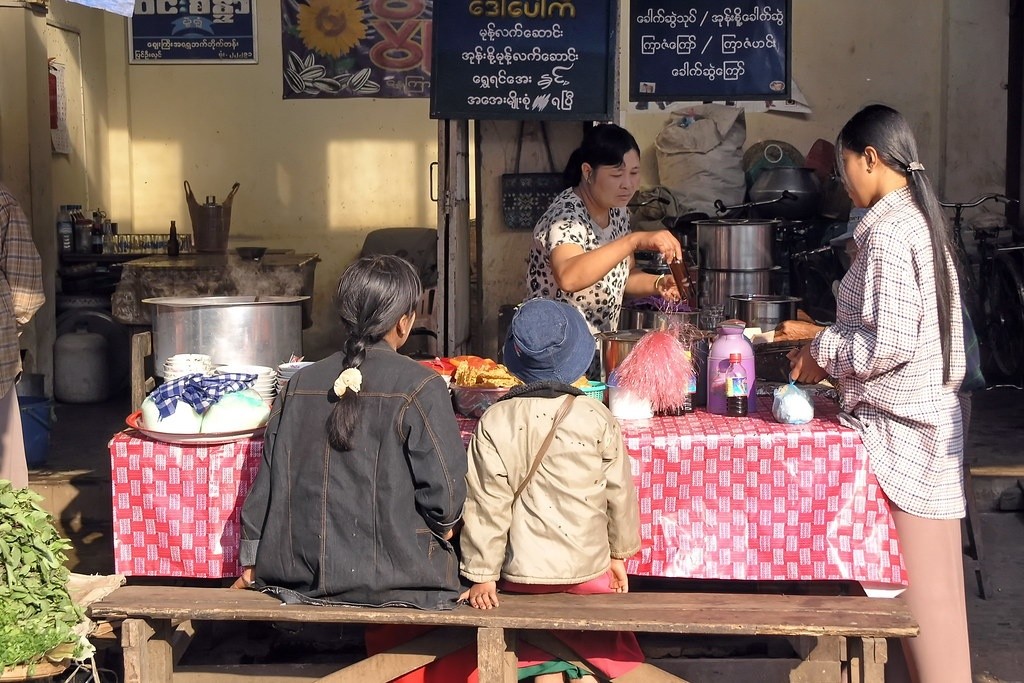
[654,274,665,290]
[239,572,256,584]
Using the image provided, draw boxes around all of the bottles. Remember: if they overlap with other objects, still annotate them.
[683,350,696,413]
[167,220,180,257]
[725,354,748,417]
[54,322,108,402]
[59,203,112,254]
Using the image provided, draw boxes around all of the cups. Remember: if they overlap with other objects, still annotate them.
[103,233,193,252]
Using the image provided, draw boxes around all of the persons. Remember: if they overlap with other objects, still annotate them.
[456,297,643,683]
[518,123,684,386]
[0,183,47,493]
[772,103,986,683]
[227,253,479,683]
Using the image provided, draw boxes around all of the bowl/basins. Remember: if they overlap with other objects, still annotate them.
[449,382,510,418]
[162,353,316,404]
[235,247,268,259]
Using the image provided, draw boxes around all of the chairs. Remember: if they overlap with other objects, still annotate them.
[362,227,440,356]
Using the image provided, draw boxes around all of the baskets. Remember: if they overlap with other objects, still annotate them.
[579,381,606,403]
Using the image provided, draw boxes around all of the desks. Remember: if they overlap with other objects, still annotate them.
[108,386,909,599]
[112,254,323,326]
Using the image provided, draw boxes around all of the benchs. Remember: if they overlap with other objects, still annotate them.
[86,582,922,683]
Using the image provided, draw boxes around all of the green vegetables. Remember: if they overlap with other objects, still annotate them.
[0,480,89,676]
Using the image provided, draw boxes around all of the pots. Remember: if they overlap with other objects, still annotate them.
[142,295,312,378]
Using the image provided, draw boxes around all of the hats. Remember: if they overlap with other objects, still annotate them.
[503,297,596,382]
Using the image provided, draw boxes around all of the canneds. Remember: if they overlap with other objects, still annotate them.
[111,234,192,254]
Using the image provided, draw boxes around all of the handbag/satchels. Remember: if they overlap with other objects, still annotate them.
[502,118,565,231]
[627,186,679,233]
[772,372,814,424]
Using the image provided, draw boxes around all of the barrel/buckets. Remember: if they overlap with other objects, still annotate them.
[17,396,54,464]
[183,180,240,253]
[594,216,802,384]
[750,167,823,214]
